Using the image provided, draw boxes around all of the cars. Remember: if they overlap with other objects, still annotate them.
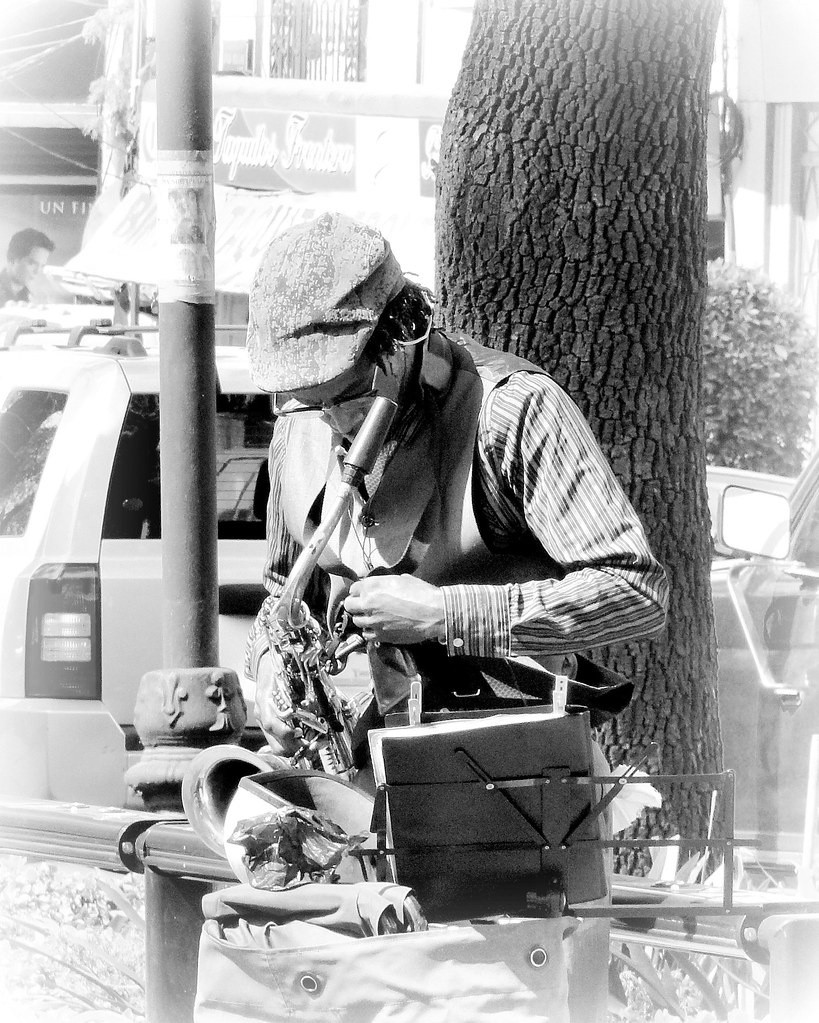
[704,444,819,829]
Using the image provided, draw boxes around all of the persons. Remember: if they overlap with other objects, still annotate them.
[0,227,56,310]
[243,212,670,1023]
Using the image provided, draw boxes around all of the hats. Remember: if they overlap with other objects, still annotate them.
[246,213,406,391]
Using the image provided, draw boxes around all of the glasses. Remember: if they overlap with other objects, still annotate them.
[274,362,379,418]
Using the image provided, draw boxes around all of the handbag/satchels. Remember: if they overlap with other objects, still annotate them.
[191,909,568,1023]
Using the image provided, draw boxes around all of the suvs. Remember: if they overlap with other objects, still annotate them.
[0,311,267,816]
